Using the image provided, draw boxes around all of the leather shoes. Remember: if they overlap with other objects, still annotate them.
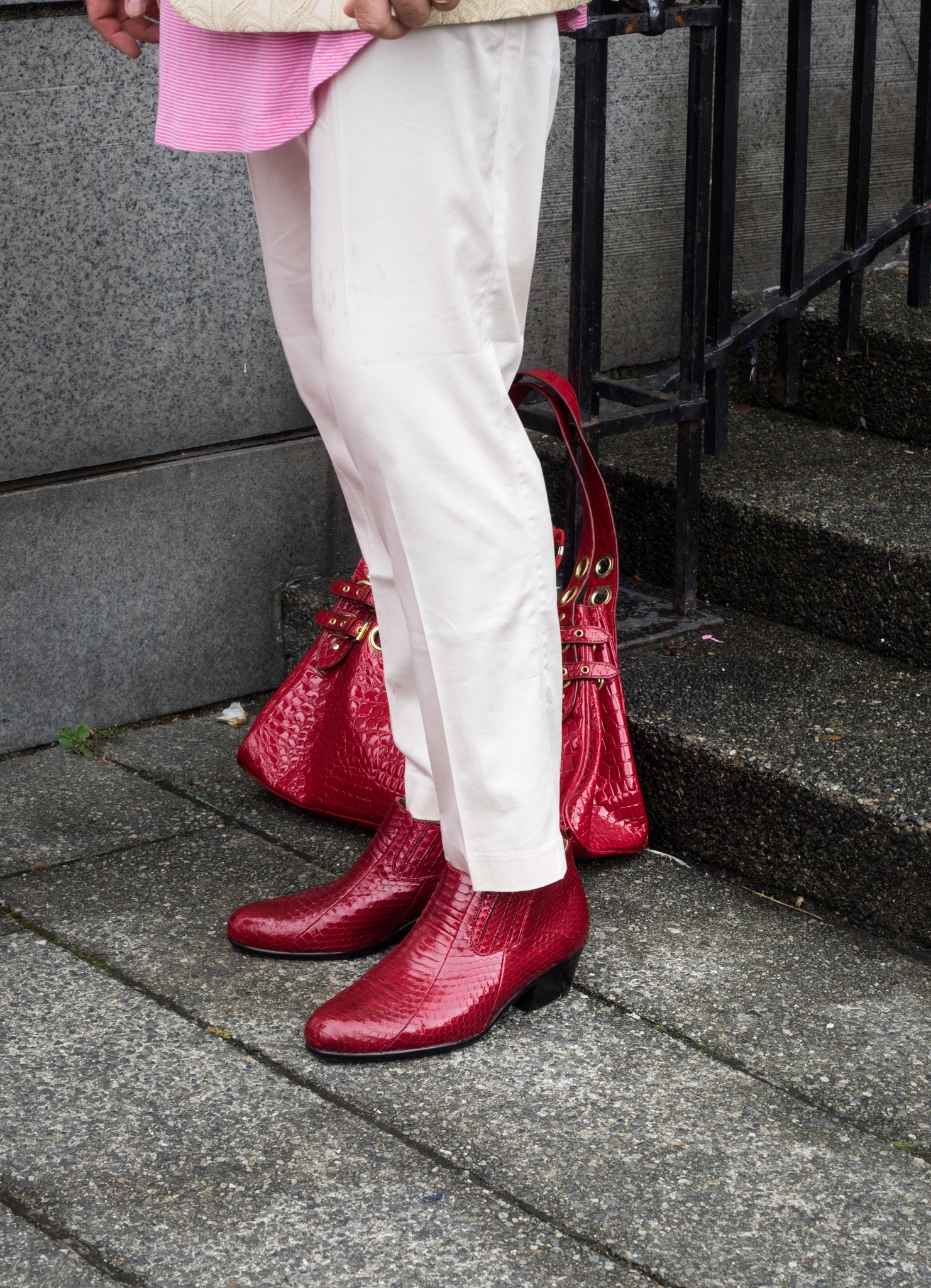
[221,798,450,962]
[302,864,590,1060]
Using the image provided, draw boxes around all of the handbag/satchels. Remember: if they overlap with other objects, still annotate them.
[239,361,654,857]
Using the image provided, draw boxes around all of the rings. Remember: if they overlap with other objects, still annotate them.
[431,0,450,3]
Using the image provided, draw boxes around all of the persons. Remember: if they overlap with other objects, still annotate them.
[84,0,594,1064]
